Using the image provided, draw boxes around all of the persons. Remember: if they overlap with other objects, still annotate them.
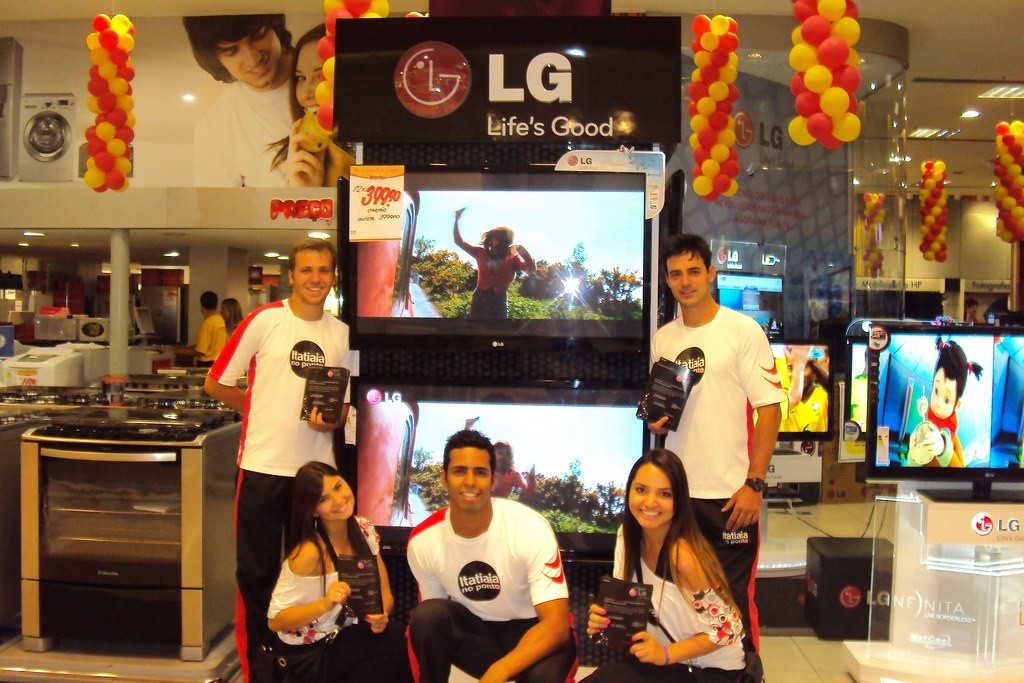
[166,291,243,368]
[648,233,786,655]
[407,430,578,683]
[182,15,327,188]
[453,208,537,319]
[204,238,350,683]
[752,345,828,432]
[266,461,406,683]
[586,448,746,683]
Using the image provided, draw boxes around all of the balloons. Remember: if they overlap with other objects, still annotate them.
[315,0,389,135]
[861,192,887,278]
[687,15,740,203]
[992,121,1024,244]
[83,14,136,193]
[787,0,861,149]
[919,161,948,263]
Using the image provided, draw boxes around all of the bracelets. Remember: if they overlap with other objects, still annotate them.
[663,646,669,665]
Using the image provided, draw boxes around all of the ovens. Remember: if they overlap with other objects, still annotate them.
[19,418,241,662]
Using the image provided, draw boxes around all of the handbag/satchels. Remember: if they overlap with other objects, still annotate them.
[265,634,328,683]
[740,649,766,683]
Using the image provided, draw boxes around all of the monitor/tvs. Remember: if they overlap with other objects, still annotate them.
[355,384,650,565]
[715,271,1024,505]
[350,163,653,357]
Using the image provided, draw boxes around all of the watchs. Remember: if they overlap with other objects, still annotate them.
[744,478,767,492]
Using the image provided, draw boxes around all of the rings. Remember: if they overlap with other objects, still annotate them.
[337,592,342,597]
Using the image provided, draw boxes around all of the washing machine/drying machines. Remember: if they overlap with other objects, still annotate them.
[16,93,78,183]
